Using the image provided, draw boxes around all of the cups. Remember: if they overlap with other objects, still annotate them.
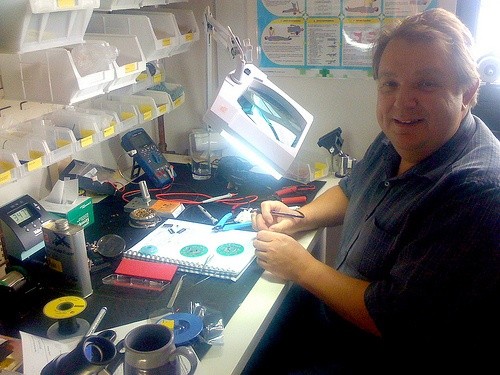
[124,323,198,375]
[188,132,212,181]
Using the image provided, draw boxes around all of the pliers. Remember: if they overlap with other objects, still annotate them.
[212,212,252,232]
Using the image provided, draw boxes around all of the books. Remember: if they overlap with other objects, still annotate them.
[123,218,257,282]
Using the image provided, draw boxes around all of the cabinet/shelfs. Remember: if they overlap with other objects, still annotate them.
[0,0,199,189]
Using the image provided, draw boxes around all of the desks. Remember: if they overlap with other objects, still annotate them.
[0,150,344,375]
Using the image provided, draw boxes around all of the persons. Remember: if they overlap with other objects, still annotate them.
[252,8,500,375]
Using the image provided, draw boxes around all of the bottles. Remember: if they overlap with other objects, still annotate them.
[41,218,94,299]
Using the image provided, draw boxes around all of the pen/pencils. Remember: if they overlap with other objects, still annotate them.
[82,307,108,341]
